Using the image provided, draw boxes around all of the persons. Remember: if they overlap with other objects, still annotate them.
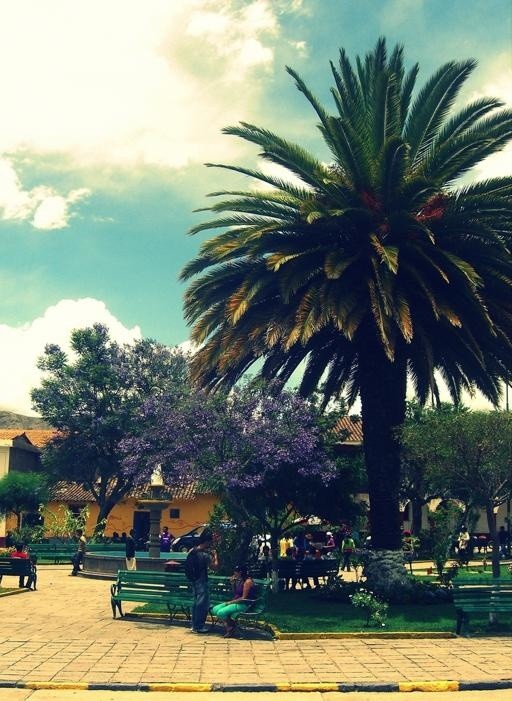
[260,532,370,589]
[160,527,175,552]
[498,526,509,559]
[12,543,34,590]
[190,531,218,633]
[69,529,87,576]
[213,564,257,637]
[112,528,150,570]
[458,527,470,566]
[403,529,411,561]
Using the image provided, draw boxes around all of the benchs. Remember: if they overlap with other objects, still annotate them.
[0,554,38,591]
[446,576,512,636]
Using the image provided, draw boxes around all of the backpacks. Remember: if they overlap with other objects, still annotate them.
[184,546,204,580]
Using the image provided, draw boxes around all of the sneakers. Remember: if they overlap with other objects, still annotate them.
[306,585,321,589]
[224,628,238,637]
[69,573,77,576]
[191,625,208,633]
[19,584,34,591]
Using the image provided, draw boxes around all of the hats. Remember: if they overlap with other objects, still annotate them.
[326,532,333,538]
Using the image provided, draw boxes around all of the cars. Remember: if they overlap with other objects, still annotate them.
[171,523,237,553]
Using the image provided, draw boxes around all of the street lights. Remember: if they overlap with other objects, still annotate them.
[493,497,499,532]
